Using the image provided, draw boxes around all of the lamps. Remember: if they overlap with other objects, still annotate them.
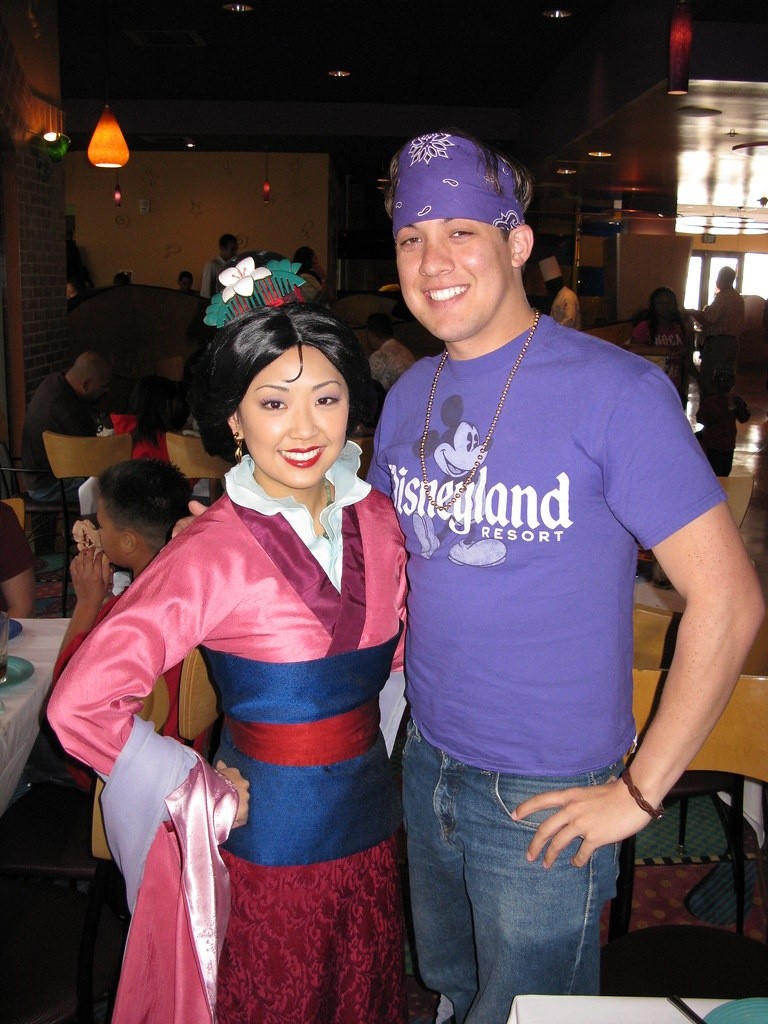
[113,170,123,208]
[87,0,130,170]
[262,150,272,205]
[667,0,693,95]
[44,132,73,164]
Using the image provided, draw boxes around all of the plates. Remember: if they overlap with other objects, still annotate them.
[0,655,35,693]
[8,618,23,640]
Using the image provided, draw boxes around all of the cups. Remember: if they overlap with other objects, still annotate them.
[0,611,10,685]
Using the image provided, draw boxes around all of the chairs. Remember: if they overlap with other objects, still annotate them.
[0,431,768,1024]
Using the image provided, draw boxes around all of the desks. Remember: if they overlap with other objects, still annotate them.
[2,615,74,816]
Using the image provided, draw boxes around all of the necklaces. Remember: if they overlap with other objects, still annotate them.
[323,476,332,540]
[419,306,541,512]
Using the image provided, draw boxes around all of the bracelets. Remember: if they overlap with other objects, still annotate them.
[621,768,665,821]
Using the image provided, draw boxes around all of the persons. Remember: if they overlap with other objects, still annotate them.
[65,235,419,437]
[46,256,410,1024]
[538,256,581,330]
[173,127,766,1024]
[18,341,214,507]
[620,268,750,475]
[0,501,35,618]
[9,456,193,810]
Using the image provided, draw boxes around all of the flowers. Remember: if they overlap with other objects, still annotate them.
[218,257,272,303]
